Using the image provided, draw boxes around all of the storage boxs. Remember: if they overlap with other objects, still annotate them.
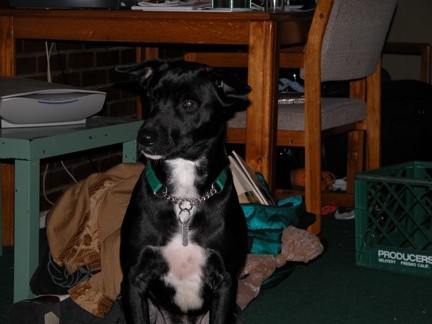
[354,161,432,280]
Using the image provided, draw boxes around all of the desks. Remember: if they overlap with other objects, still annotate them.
[0,8,366,245]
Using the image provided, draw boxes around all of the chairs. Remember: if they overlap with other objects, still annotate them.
[184,0,398,234]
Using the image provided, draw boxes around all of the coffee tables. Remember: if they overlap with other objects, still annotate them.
[0,118,144,304]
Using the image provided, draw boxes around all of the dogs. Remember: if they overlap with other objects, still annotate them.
[107,59,254,324]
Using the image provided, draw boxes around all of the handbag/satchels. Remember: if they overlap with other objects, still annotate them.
[239,195,305,255]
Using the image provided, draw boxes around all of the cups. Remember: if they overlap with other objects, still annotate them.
[263,0,285,12]
